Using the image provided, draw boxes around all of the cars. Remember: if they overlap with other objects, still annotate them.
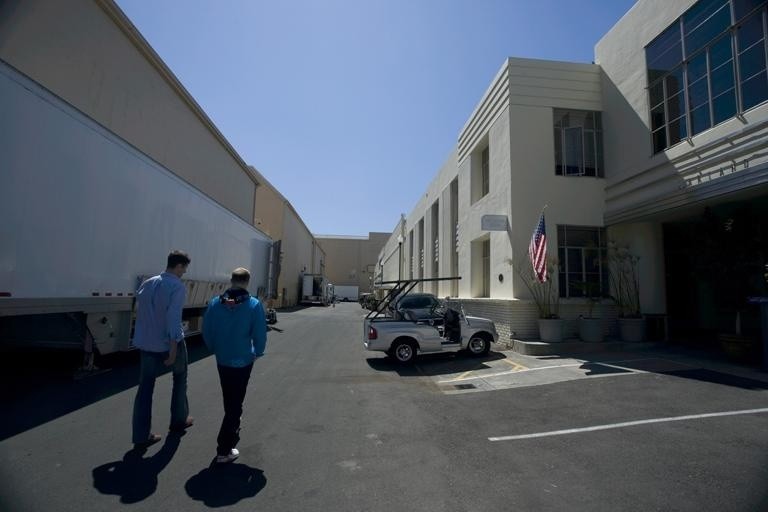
[359,292,500,366]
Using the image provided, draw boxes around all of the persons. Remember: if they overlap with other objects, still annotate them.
[129,247,194,448]
[199,265,268,465]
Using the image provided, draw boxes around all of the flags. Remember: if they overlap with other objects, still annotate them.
[527,209,548,285]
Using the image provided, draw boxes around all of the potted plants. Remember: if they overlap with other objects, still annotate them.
[503,242,647,343]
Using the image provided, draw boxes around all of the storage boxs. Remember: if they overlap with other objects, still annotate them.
[181,316,204,331]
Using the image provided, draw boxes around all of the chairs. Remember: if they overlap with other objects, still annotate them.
[404,310,434,325]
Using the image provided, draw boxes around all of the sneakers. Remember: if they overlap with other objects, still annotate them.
[216,448,239,462]
[134,433,161,447]
[169,416,193,431]
